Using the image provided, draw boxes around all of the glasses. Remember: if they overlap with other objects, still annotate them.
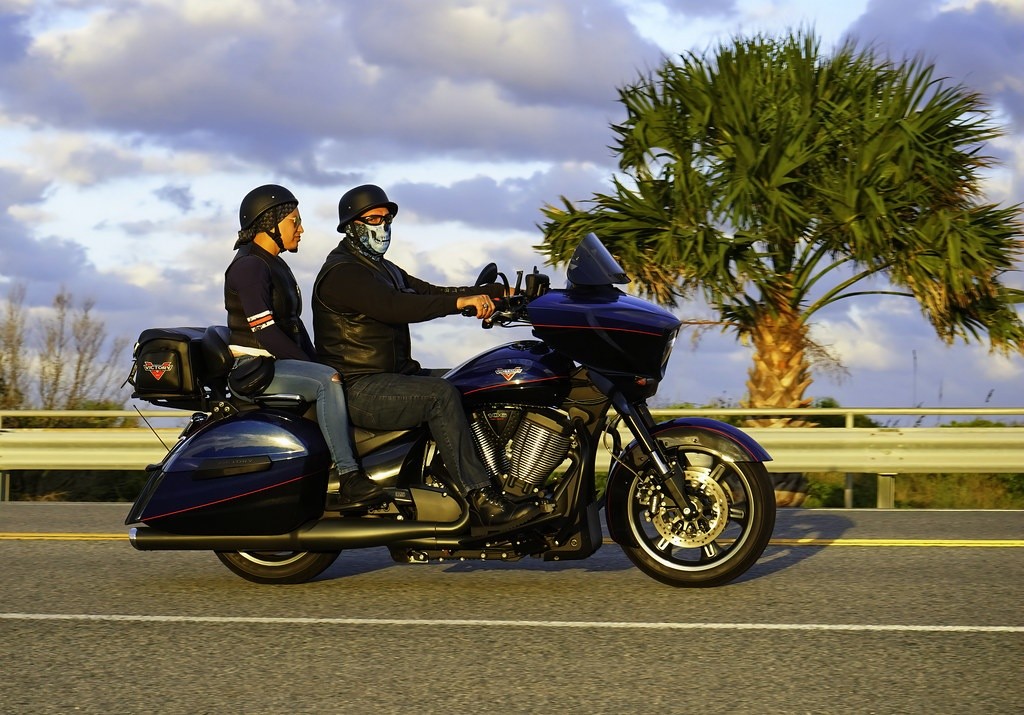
[355,213,393,226]
[278,216,301,228]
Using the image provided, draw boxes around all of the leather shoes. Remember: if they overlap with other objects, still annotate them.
[340,470,385,502]
[466,486,540,536]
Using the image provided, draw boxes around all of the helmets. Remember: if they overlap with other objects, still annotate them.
[239,184,299,230]
[336,184,399,233]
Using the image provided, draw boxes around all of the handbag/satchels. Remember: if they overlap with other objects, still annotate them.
[229,355,275,398]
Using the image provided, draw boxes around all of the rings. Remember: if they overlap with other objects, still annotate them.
[482,303,487,309]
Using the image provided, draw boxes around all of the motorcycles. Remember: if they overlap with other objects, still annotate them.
[124,231,778,588]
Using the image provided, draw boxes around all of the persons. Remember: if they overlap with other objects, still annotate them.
[224,184,384,504]
[312,184,542,537]
